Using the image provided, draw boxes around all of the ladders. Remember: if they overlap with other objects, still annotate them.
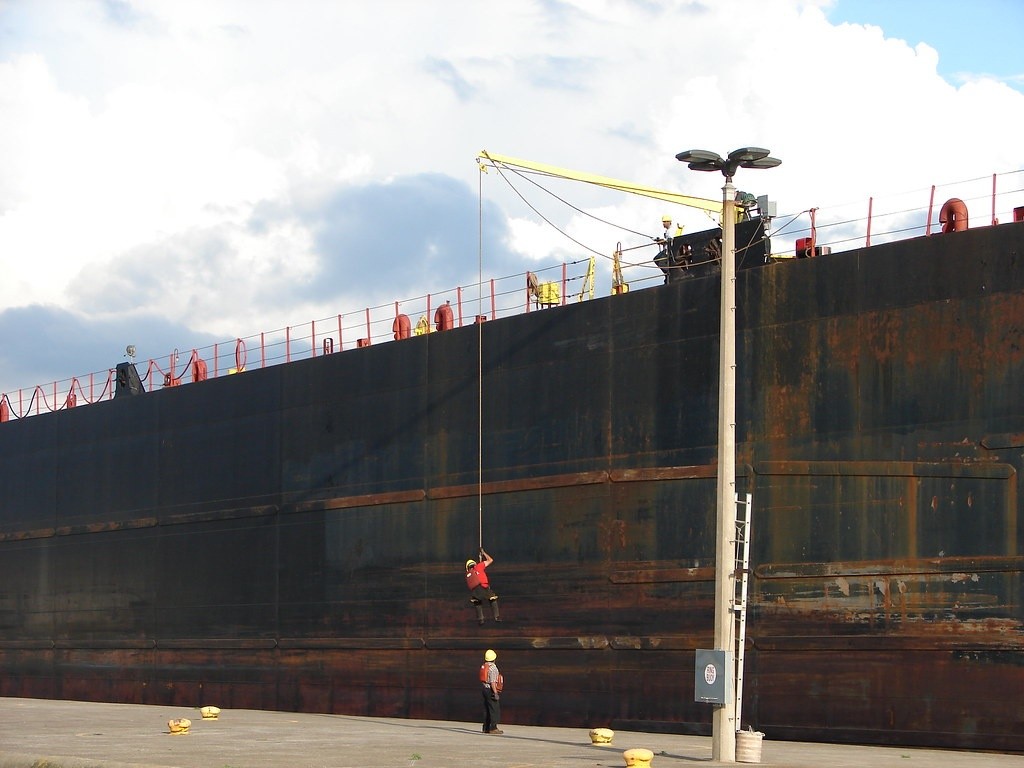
[732,488,754,733]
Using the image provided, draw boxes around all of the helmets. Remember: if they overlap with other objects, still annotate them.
[485,649,497,661]
[662,215,672,222]
[466,560,477,572]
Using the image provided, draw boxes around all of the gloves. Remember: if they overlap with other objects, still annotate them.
[491,693,500,700]
[480,548,485,556]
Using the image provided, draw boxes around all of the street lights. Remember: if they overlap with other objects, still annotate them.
[670,147,784,761]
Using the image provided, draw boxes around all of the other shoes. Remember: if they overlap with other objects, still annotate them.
[479,619,487,625]
[664,277,667,282]
[494,617,502,623]
[483,727,503,734]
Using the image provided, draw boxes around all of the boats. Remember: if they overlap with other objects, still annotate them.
[1,164,1024,761]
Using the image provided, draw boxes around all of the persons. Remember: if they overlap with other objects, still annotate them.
[465,547,502,626]
[654,214,674,283]
[479,649,504,734]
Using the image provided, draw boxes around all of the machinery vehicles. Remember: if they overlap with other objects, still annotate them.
[476,149,776,284]
